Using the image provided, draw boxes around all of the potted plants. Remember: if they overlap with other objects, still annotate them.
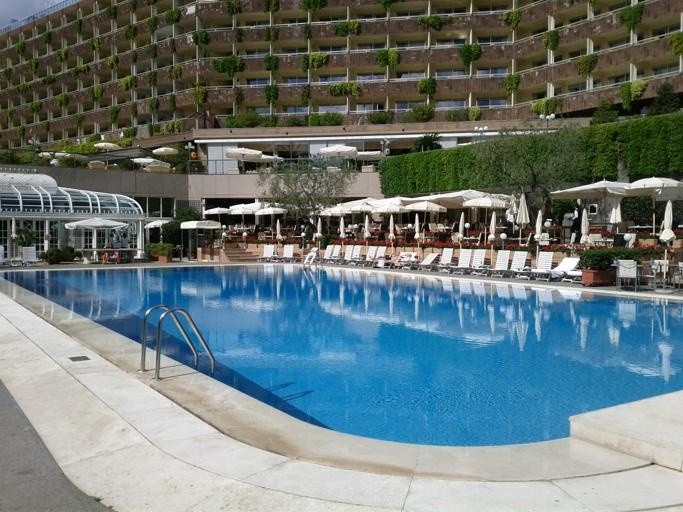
[147,243,174,263]
[636,233,683,249]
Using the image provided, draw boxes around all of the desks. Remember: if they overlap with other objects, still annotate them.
[594,240,614,248]
[527,226,602,246]
[627,225,683,233]
[73,248,137,264]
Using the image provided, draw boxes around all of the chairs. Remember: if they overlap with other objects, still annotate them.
[468,248,490,276]
[417,252,440,271]
[345,221,535,245]
[611,258,683,293]
[530,251,555,280]
[437,247,454,274]
[450,248,473,274]
[357,245,386,266]
[458,281,582,304]
[387,251,421,269]
[258,244,342,264]
[618,220,636,233]
[342,246,365,265]
[0,244,43,269]
[618,298,637,323]
[550,257,581,282]
[489,249,530,280]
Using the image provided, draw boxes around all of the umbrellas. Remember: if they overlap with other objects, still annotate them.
[38,142,178,168]
[143,219,169,242]
[580,208,589,249]
[534,209,542,267]
[659,199,676,290]
[550,177,683,238]
[319,144,358,172]
[64,217,129,262]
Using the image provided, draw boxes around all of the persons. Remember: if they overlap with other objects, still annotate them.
[101,229,128,265]
[569,203,581,246]
[224,147,284,174]
